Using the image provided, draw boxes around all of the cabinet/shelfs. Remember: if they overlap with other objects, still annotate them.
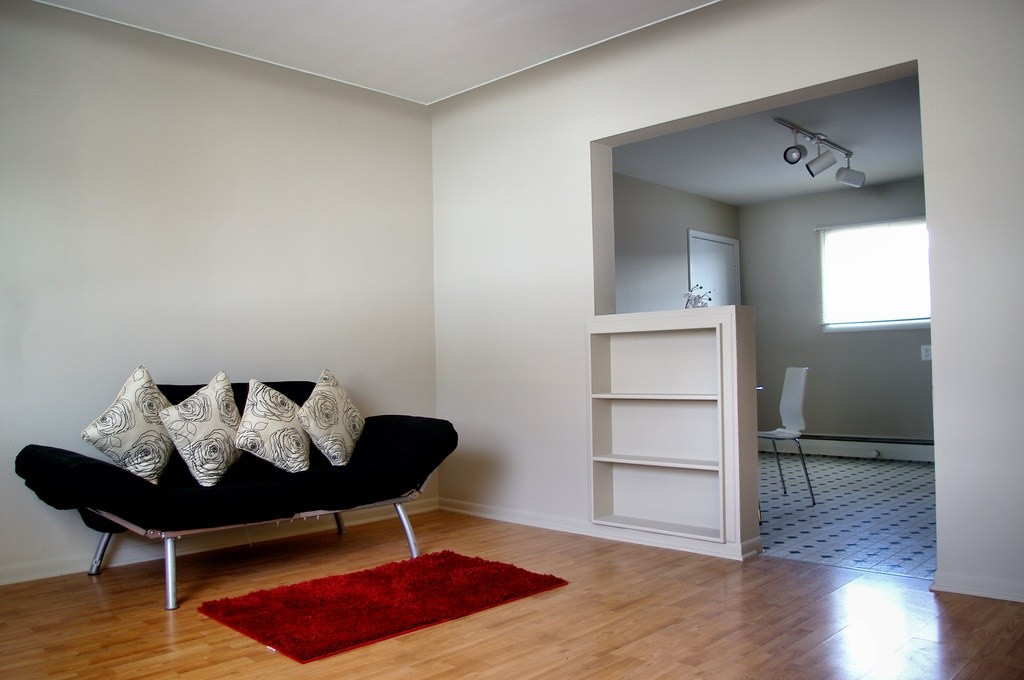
[587,322,726,546]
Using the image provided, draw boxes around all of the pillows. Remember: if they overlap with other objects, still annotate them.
[159,370,245,487]
[80,363,175,485]
[234,377,310,473]
[296,369,365,467]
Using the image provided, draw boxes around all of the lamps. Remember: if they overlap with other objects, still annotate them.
[783,131,808,166]
[805,144,837,178]
[836,157,866,189]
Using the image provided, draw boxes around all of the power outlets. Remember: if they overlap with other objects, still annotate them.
[920,344,931,361]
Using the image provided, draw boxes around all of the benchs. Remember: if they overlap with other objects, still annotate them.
[15,384,460,611]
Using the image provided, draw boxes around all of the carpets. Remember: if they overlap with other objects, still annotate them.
[197,548,570,665]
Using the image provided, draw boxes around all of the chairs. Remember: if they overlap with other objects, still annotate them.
[757,365,816,506]
